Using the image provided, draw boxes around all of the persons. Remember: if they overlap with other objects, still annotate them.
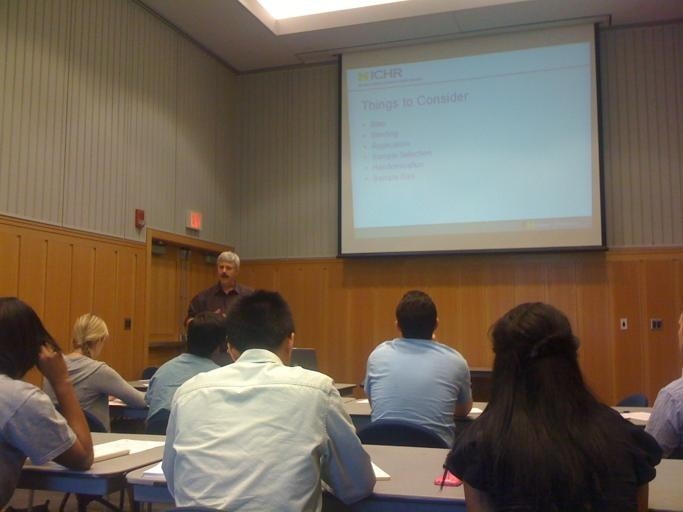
[185,251,255,323]
[441,302,662,512]
[0,298,94,512]
[363,291,473,448]
[44,312,227,433]
[162,290,375,512]
[644,310,683,458]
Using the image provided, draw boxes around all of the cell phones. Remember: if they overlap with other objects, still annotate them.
[435,470,463,486]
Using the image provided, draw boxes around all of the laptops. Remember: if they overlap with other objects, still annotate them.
[290,348,317,371]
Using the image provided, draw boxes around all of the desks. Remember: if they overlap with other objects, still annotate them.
[14,382,683,511]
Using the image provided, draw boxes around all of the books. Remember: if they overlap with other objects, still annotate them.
[357,399,369,404]
[371,462,392,481]
[619,410,651,426]
[143,460,166,477]
[468,407,483,416]
[93,441,130,462]
